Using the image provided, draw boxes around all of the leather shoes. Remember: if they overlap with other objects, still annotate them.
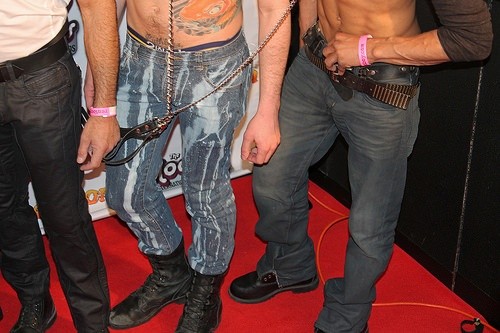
[9,296,57,333]
[229,264,319,303]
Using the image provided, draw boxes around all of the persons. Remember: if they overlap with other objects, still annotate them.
[228,0,494,333]
[84,0,291,333]
[0,0,120,333]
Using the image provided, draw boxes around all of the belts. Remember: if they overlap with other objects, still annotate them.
[0,29,70,81]
[305,24,420,100]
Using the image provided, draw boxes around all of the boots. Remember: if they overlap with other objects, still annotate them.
[108,235,230,333]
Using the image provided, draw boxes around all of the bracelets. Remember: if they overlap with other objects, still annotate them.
[89,106,117,118]
[358,34,373,67]
